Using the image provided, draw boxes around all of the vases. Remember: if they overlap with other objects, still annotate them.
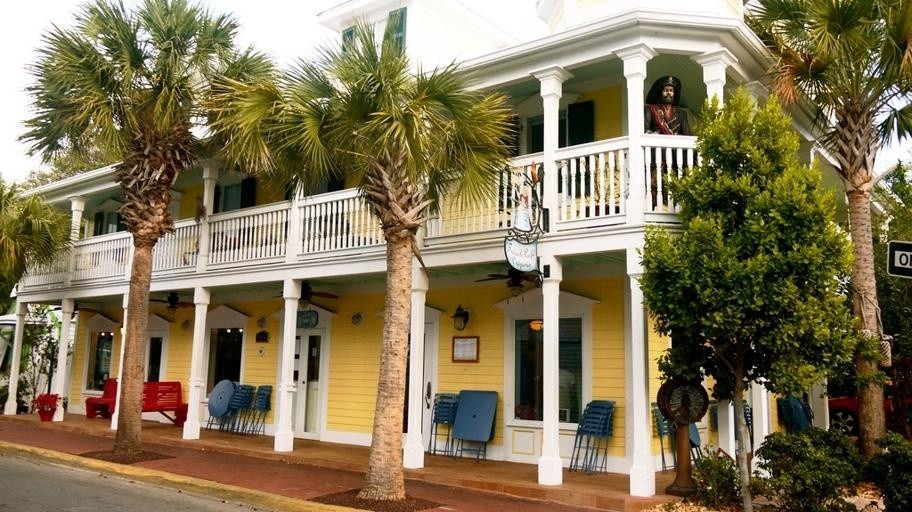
[37,408,56,422]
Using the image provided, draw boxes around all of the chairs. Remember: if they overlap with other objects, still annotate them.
[651,402,677,474]
[205,384,273,437]
[426,391,499,462]
[567,400,618,475]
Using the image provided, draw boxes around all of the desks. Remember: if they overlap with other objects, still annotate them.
[690,422,705,468]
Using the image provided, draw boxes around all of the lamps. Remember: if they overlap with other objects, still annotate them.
[509,271,525,299]
[302,280,311,307]
[451,305,470,332]
[165,290,178,321]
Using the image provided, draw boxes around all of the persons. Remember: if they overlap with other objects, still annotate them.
[644,74,694,211]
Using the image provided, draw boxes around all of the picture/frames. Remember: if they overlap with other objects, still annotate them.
[452,335,480,362]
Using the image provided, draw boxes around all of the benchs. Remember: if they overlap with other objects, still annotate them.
[84,378,195,427]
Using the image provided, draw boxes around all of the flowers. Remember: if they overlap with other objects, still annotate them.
[33,393,61,410]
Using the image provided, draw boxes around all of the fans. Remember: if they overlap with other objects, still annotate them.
[149,293,195,312]
[299,280,340,314]
[474,268,541,285]
